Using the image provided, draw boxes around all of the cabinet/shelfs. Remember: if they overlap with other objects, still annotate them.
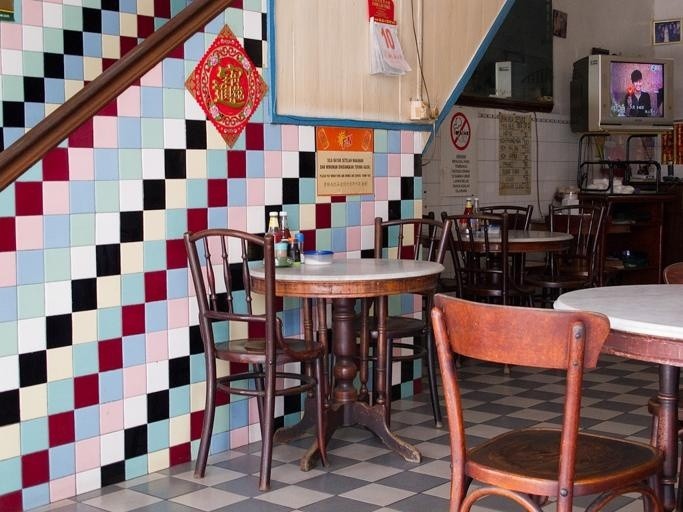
[555,192,677,284]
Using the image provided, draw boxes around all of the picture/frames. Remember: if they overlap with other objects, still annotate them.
[651,18,683,46]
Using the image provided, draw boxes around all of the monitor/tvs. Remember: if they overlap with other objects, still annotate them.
[569,53,673,133]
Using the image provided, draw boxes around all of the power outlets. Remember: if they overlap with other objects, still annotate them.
[410,101,430,121]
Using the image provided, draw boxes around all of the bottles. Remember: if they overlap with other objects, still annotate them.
[296,233,303,250]
[275,241,289,265]
[279,211,290,237]
[290,238,299,260]
[459,198,472,241]
[470,198,480,239]
[267,212,280,240]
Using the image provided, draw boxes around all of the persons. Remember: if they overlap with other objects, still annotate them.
[624,70,651,117]
[657,88,663,117]
